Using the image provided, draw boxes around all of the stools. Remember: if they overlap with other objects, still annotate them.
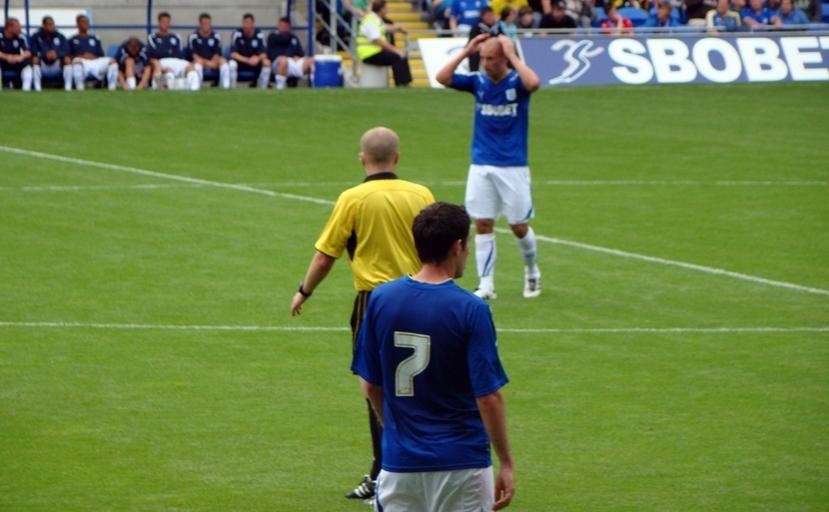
[359,64,390,88]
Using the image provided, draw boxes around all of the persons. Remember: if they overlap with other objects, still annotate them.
[228,13,273,90]
[351,202,515,512]
[146,13,200,93]
[114,38,152,91]
[0,18,33,91]
[437,32,542,303]
[291,126,438,502]
[29,17,73,93]
[268,18,316,91]
[68,16,119,92]
[185,13,230,92]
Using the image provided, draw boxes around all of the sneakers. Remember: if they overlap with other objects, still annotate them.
[344,474,387,501]
[466,285,498,300]
[523,266,543,299]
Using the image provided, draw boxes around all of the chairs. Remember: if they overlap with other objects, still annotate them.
[422,0,784,36]
[0,54,300,89]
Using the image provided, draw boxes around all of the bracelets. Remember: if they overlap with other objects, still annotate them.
[299,282,312,297]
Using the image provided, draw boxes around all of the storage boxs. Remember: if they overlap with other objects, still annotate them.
[310,54,344,88]
